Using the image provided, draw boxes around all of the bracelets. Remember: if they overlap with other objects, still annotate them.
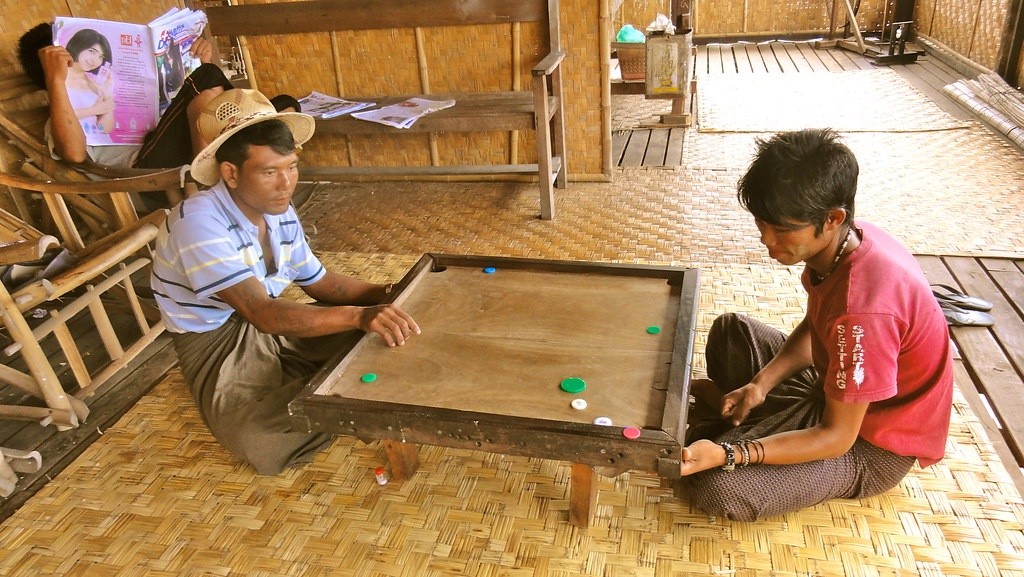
[386,283,393,294]
[728,439,765,468]
[717,441,736,472]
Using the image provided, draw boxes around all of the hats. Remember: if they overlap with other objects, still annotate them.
[190,88,315,186]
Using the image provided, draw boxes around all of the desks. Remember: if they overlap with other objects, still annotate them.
[286,252,702,529]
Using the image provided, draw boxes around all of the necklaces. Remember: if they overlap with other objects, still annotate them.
[814,227,852,287]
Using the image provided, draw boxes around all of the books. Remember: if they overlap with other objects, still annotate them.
[50,6,209,146]
[295,90,456,129]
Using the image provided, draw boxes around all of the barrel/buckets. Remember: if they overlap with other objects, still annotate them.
[646,28,693,99]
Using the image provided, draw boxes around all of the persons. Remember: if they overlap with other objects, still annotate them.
[146,119,421,477]
[678,127,954,524]
[65,28,114,144]
[18,17,304,202]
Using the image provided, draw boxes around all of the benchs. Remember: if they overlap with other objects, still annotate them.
[184,0,569,220]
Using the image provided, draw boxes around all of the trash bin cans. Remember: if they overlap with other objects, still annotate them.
[616,25,646,80]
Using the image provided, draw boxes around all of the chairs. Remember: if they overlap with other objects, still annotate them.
[0,73,183,244]
[0,164,192,433]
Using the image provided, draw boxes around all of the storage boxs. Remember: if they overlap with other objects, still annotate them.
[611,42,645,80]
